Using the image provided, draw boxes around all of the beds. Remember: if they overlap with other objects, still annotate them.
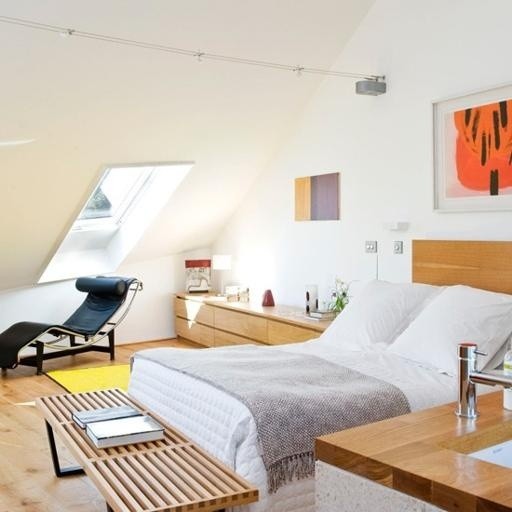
[129,240,512,512]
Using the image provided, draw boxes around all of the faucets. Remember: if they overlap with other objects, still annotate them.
[458,344,509,408]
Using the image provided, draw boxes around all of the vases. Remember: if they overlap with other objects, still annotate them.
[334,310,342,320]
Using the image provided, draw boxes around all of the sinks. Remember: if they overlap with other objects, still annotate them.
[466,439,512,470]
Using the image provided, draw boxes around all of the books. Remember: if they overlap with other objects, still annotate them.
[84,413,166,448]
[72,403,144,430]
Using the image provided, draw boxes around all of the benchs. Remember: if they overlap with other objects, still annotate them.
[37,389,260,512]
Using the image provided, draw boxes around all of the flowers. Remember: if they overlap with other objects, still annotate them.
[323,279,352,312]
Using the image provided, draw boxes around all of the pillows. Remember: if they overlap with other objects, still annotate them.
[76,277,127,296]
[320,277,445,353]
[386,285,512,384]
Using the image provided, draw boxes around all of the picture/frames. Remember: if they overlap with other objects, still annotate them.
[432,83,512,214]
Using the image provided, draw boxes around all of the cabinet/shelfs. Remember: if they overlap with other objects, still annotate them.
[268,317,324,349]
[215,306,268,349]
[174,291,215,348]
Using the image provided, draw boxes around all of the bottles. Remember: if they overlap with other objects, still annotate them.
[502,348,512,412]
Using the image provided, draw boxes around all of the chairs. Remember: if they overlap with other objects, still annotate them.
[0,276,143,375]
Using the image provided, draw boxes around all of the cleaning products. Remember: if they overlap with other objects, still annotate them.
[504,350,512,410]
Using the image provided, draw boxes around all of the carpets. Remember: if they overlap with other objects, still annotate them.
[43,364,133,396]
